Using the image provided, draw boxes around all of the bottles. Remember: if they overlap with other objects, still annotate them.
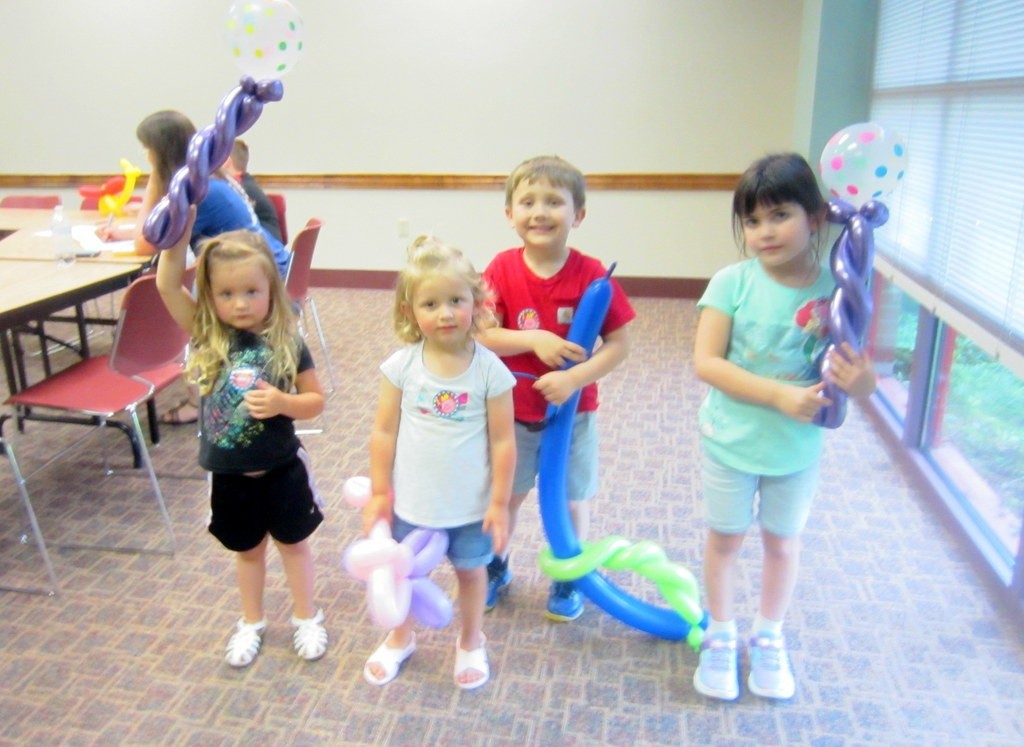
[51,205,73,265]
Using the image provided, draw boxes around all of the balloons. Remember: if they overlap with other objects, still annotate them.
[143,0,305,250]
[813,121,908,430]
[541,535,704,655]
[539,262,709,643]
[343,476,452,629]
[78,158,143,219]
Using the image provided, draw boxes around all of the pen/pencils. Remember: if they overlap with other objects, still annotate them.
[106,214,114,229]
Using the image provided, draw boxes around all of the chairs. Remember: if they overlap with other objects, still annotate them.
[1,257,203,558]
[0,195,62,210]
[81,196,143,210]
[264,194,336,436]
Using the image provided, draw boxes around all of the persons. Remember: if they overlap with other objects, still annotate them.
[95,110,267,426]
[691,152,876,700]
[156,203,325,669]
[362,235,518,688]
[220,140,283,244]
[469,157,635,622]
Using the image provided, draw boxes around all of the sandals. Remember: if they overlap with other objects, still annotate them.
[291,608,330,662]
[225,614,268,667]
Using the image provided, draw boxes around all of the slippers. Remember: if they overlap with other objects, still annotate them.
[454,634,490,689]
[363,628,416,686]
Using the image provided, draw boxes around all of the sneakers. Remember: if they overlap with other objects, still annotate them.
[693,629,739,700]
[479,553,511,613]
[546,580,584,622]
[747,630,796,699]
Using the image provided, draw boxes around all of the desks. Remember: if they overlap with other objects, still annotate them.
[0,205,160,470]
